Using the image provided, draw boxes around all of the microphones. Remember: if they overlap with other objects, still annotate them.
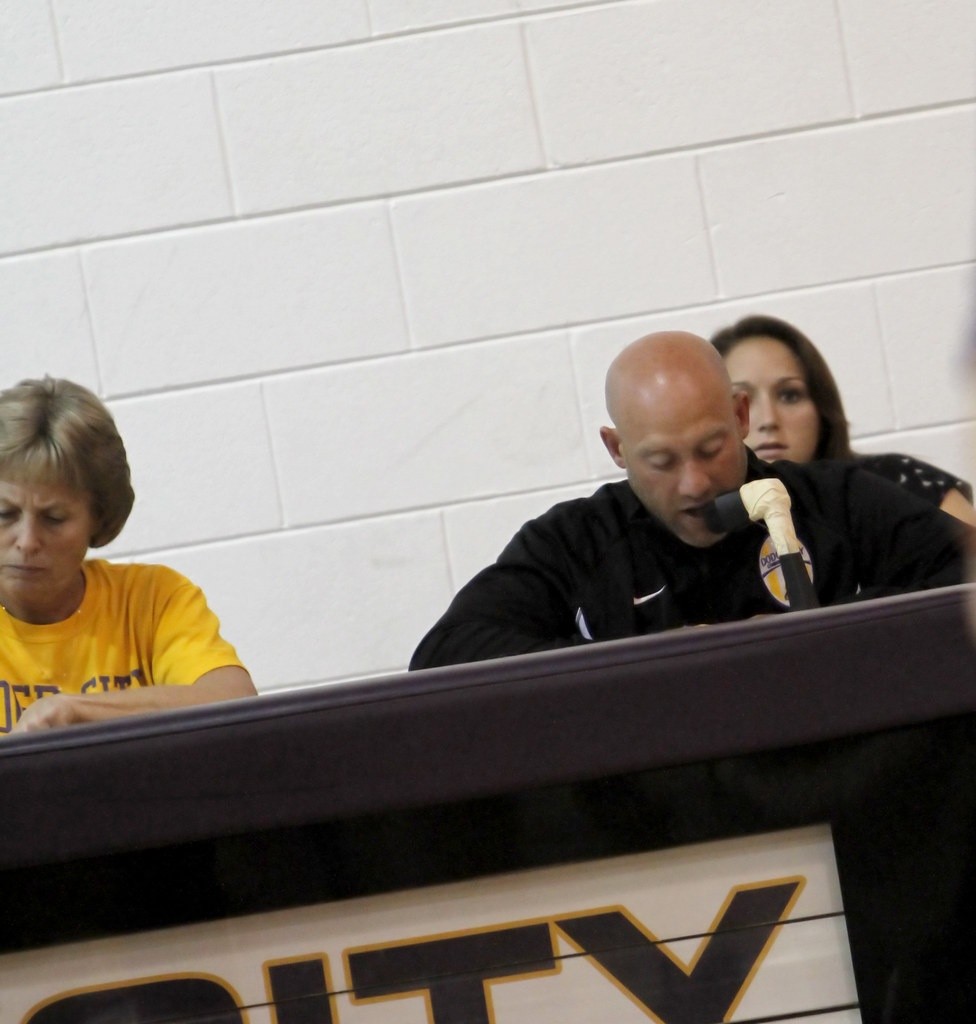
[702,478,791,536]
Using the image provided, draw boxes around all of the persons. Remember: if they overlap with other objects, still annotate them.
[408,332,976,673]
[1,372,259,735]
[711,315,976,527]
